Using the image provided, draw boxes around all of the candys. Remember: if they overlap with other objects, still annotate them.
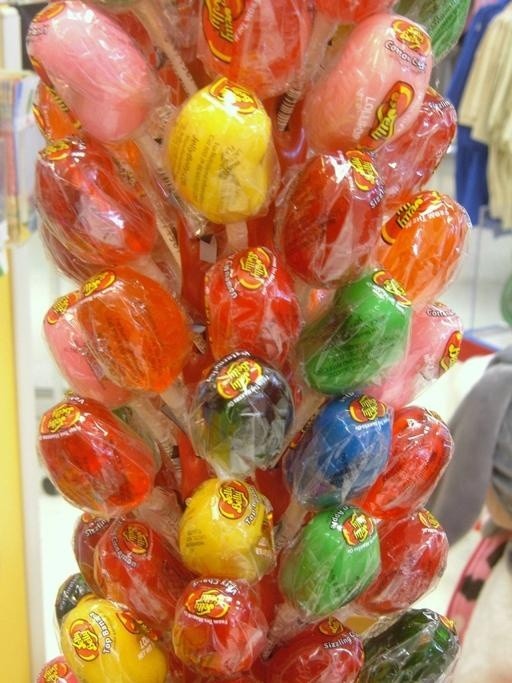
[25,0,474,683]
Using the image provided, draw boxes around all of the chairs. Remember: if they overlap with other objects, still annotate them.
[424,361,512,574]
